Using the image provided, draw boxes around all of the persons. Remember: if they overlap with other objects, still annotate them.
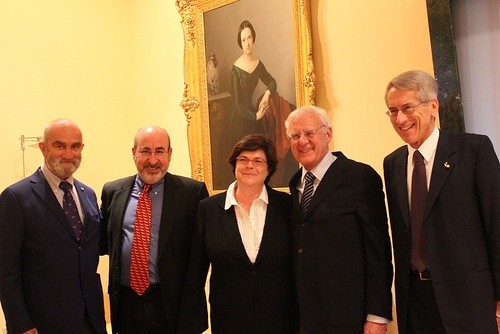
[286,105,392,334]
[0,118,107,334]
[97,126,209,334]
[382,69,500,334]
[198,134,292,334]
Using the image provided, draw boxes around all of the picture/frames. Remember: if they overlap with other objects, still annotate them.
[175,0,316,198]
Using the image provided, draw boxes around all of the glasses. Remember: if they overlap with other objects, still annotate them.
[288,125,325,142]
[235,156,269,166]
[386,101,426,118]
[136,148,169,156]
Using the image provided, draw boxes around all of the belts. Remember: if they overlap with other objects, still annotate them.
[409,269,432,281]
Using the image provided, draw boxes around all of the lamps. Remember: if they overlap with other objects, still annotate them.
[19,136,42,150]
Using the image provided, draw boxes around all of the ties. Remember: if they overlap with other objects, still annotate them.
[129,184,152,297]
[300,172,316,217]
[411,150,428,273]
[59,180,83,244]
